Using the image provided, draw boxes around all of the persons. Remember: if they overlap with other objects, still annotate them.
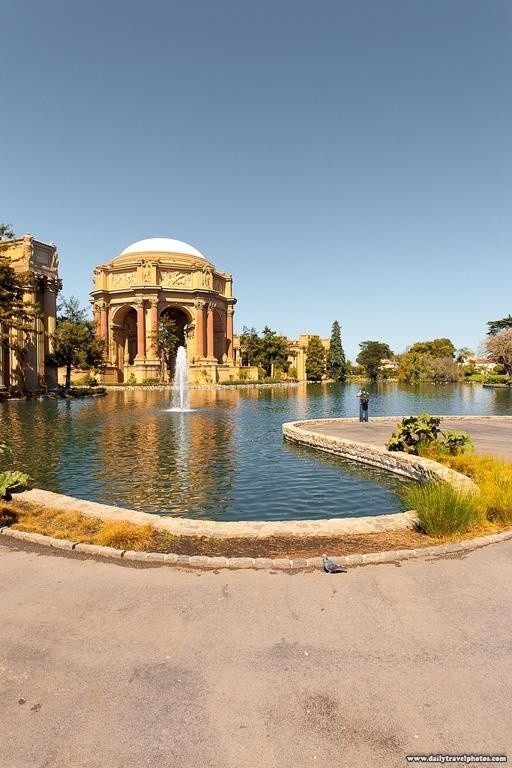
[356,386,369,424]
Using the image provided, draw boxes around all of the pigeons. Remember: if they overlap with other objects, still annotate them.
[322,556,348,574]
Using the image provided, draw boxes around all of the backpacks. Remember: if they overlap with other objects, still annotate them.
[360,392,368,404]
